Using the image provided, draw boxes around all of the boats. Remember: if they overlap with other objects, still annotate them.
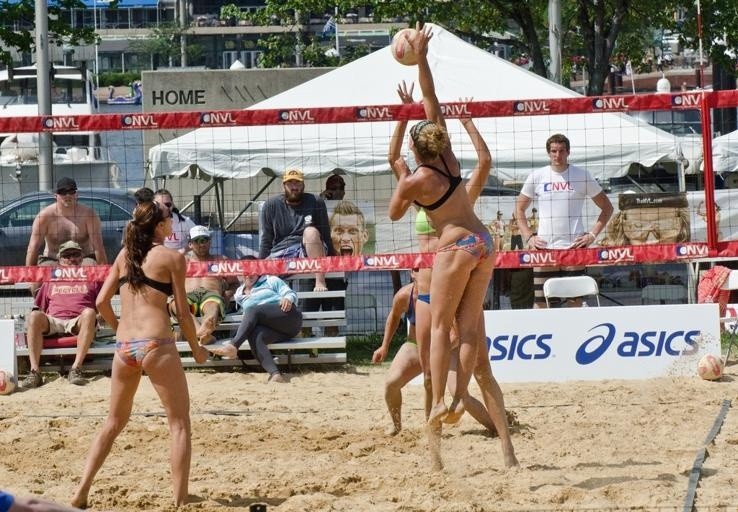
[626,102,705,179]
[105,83,142,105]
[0,48,119,200]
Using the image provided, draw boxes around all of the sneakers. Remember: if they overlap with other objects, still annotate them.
[23,373,42,388]
[68,369,85,385]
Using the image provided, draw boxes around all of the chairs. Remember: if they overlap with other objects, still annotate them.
[699,268,738,365]
[543,275,601,309]
[642,285,689,305]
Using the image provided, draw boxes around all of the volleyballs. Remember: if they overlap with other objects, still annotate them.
[392,29,429,65]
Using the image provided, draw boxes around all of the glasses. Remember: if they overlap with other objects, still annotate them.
[620,216,688,242]
[195,237,208,243]
[57,188,75,195]
[62,252,81,258]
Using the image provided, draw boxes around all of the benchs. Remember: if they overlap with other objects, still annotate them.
[17,271,347,371]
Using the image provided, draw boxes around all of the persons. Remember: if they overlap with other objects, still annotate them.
[515,132,614,307]
[492,200,721,255]
[390,78,523,472]
[153,189,197,256]
[210,255,302,384]
[371,254,500,436]
[167,225,240,344]
[22,242,104,388]
[389,21,494,427]
[258,172,345,336]
[70,201,212,507]
[25,177,107,314]
[0,490,91,511]
[328,202,367,255]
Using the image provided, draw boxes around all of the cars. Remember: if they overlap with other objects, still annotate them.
[0,188,141,266]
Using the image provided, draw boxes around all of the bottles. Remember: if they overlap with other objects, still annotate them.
[12,313,27,347]
[580,301,589,307]
[704,296,713,303]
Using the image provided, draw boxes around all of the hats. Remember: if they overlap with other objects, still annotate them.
[284,169,303,182]
[189,225,210,239]
[59,241,82,253]
[57,177,77,190]
